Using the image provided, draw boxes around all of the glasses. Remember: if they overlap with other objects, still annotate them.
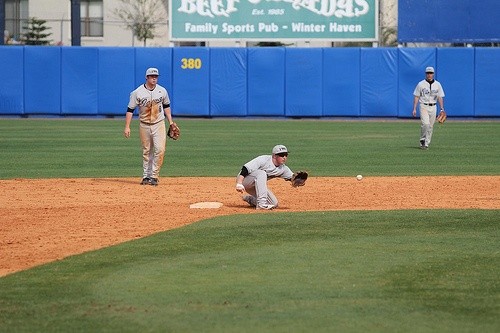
[425,72,434,74]
[274,153,288,157]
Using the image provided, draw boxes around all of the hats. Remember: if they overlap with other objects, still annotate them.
[425,67,435,73]
[272,144,288,154]
[145,67,159,77]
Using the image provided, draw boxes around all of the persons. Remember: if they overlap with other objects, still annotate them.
[413,67,445,148]
[4,30,17,44]
[236,145,300,210]
[125,68,178,185]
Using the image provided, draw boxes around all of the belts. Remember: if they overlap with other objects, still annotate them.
[423,103,436,106]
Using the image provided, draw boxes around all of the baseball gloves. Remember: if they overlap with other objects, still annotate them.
[291,171,308,187]
[167,123,180,140]
[437,111,447,124]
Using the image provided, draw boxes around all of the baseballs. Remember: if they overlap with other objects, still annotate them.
[356,174,362,181]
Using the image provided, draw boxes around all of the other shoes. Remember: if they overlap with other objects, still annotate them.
[242,195,256,206]
[419,136,430,149]
[150,178,159,185]
[140,178,150,185]
[256,203,274,210]
